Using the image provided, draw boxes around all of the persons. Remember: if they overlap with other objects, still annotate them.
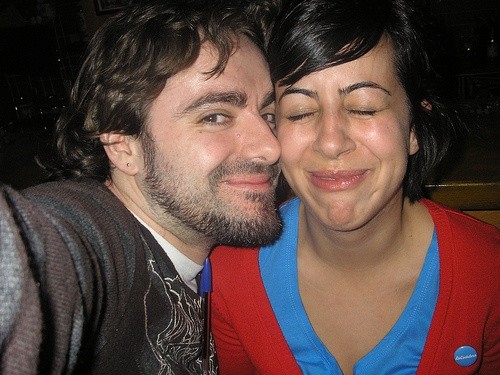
[0,0,281,375]
[207,0,500,375]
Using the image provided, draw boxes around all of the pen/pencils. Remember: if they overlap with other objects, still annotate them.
[200,257,214,374]
